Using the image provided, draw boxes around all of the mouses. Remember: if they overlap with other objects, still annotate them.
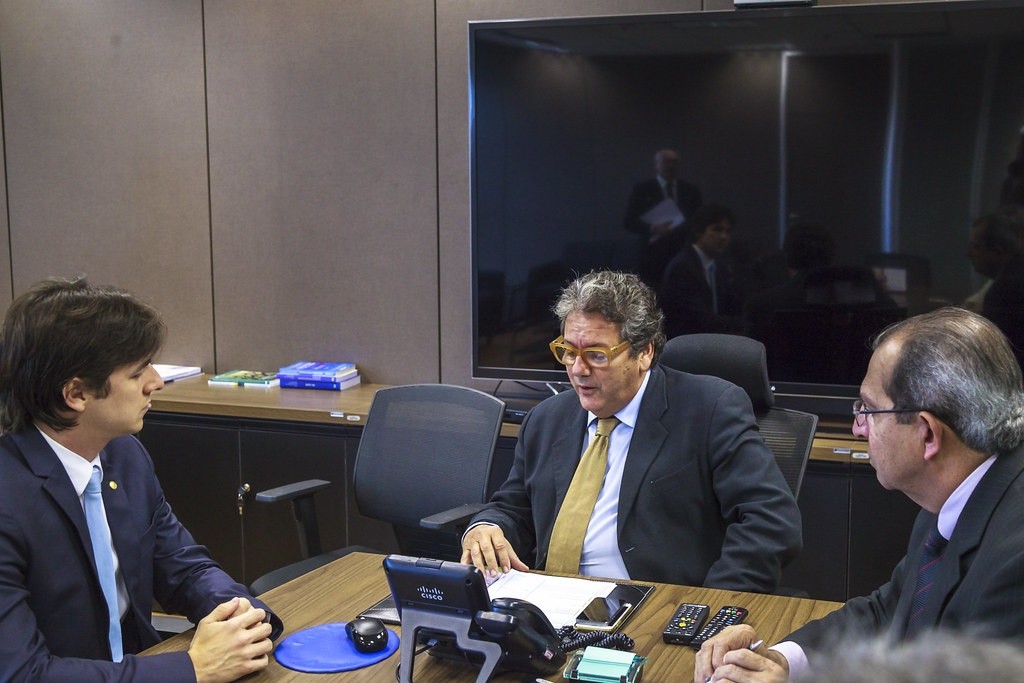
[345,616,388,652]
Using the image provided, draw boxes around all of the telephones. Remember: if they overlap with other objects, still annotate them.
[381,556,566,677]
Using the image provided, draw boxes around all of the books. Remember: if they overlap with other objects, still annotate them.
[277,361,360,390]
[208,370,280,387]
[151,365,205,383]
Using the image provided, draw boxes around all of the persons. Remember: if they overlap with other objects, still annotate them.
[661,204,745,339]
[624,150,702,291]
[461,271,804,595]
[694,306,1024,683]
[0,274,285,683]
[965,126,1024,368]
[748,222,907,385]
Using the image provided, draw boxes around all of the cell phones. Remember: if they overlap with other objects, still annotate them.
[574,603,632,632]
[576,597,625,627]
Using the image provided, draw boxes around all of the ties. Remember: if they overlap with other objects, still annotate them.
[708,265,714,309]
[545,416,622,575]
[665,182,674,200]
[83,465,123,664]
[901,524,950,647]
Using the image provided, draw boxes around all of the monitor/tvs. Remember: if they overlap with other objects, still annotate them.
[467,0,1023,399]
[383,553,508,666]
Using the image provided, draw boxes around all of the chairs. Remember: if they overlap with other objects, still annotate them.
[659,334,818,504]
[250,383,506,594]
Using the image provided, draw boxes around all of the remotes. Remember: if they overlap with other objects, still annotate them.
[689,606,748,651]
[663,603,709,643]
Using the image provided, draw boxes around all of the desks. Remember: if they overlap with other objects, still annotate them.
[133,366,923,602]
[121,545,847,683]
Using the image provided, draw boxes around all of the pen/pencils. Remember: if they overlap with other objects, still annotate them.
[706,639,764,683]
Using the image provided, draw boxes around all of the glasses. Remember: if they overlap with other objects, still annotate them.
[852,399,953,429]
[549,335,631,369]
